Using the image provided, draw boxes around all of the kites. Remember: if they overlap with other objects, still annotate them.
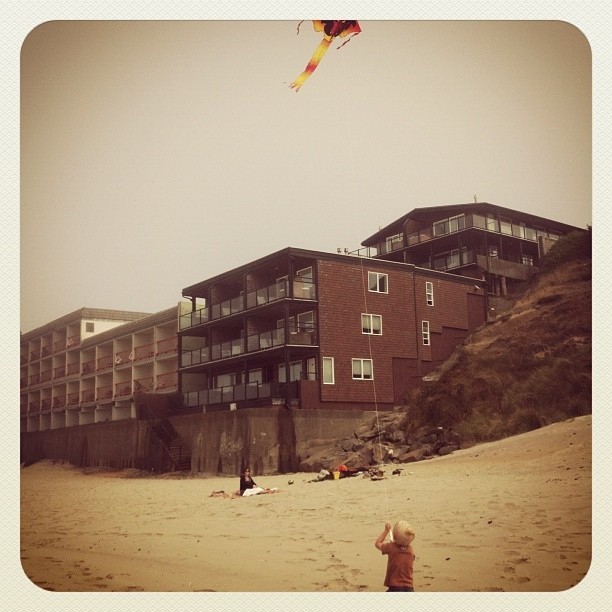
[287,20,362,95]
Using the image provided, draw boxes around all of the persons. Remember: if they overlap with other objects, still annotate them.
[377,521,416,592]
[238,467,280,501]
